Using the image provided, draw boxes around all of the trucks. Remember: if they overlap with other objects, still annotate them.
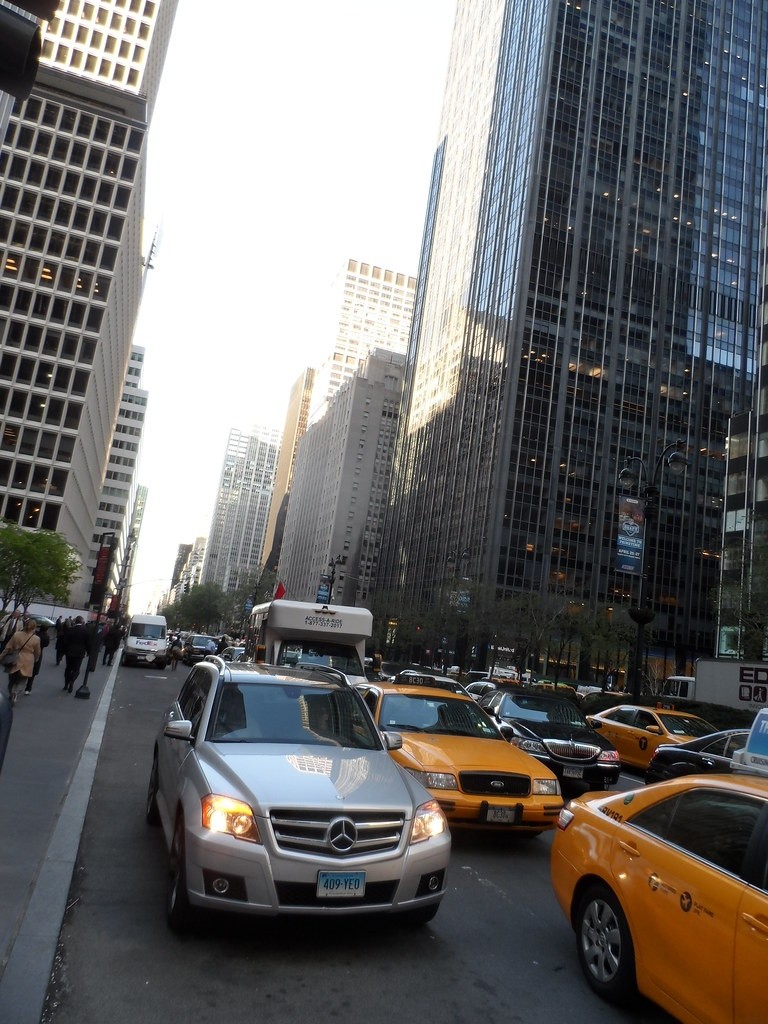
[243,598,374,686]
[120,612,168,670]
[659,657,768,712]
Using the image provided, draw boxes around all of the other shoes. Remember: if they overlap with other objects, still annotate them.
[67,686,73,693]
[63,684,68,690]
[102,662,107,665]
[56,661,60,665]
[24,690,31,695]
[10,696,19,706]
[107,663,113,666]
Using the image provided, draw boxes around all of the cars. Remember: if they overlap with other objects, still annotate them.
[353,675,563,847]
[167,629,207,648]
[551,708,768,1024]
[645,728,750,783]
[586,704,744,774]
[0,612,56,633]
[480,686,621,790]
[365,656,633,702]
[216,647,245,663]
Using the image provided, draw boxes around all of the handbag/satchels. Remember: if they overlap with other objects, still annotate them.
[2,653,19,667]
[60,627,79,649]
[166,656,171,665]
[173,646,183,658]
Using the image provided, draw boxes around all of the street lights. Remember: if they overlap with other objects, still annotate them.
[326,553,347,608]
[113,529,137,627]
[618,437,691,702]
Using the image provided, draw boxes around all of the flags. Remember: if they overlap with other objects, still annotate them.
[273,581,286,600]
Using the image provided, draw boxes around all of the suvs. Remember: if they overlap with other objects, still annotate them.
[145,653,452,941]
[182,635,219,665]
[86,621,107,633]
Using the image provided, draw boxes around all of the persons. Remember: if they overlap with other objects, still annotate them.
[54,615,128,667]
[0,619,41,703]
[55,615,89,692]
[204,634,247,660]
[441,663,447,676]
[0,608,23,656]
[168,635,186,671]
[0,665,13,777]
[22,623,50,695]
[20,611,33,628]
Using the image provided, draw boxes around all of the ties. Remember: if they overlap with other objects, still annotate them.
[7,619,14,636]
